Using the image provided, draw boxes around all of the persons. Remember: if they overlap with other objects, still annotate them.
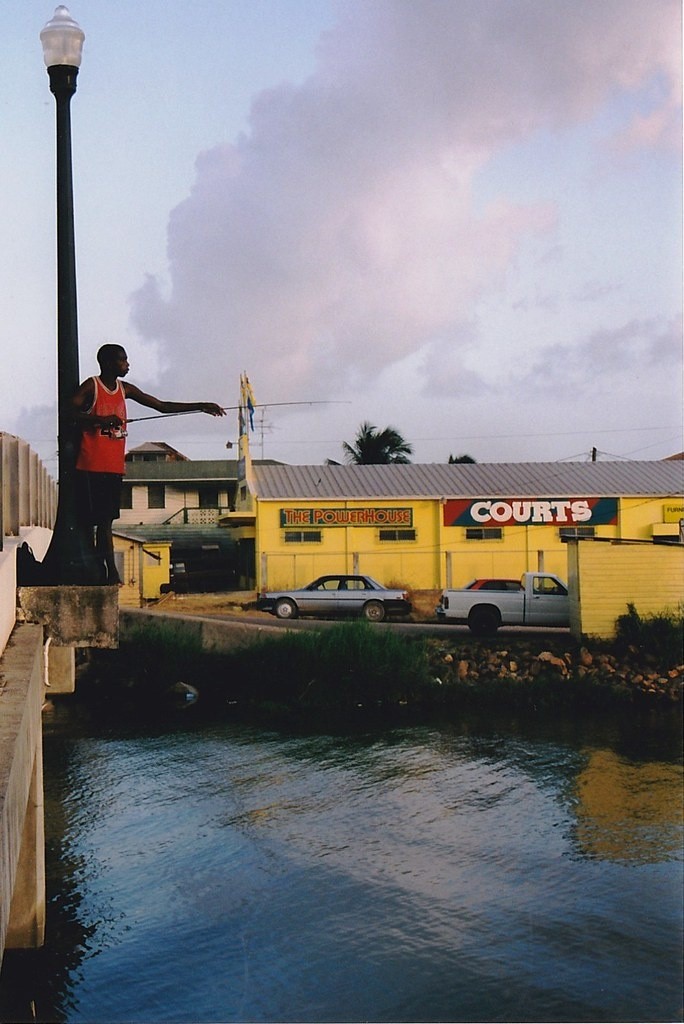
[72,344,226,586]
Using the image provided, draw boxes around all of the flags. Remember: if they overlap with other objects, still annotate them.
[239,375,257,432]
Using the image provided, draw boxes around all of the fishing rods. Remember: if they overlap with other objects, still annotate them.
[92,399,351,439]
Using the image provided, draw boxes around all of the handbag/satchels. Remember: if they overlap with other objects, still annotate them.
[17,542,43,586]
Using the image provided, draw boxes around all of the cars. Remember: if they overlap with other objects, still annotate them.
[257,574,414,623]
[464,578,522,591]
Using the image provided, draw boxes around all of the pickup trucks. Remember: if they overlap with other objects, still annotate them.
[435,569,571,635]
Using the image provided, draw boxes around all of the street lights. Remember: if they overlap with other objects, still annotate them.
[38,6,110,587]
